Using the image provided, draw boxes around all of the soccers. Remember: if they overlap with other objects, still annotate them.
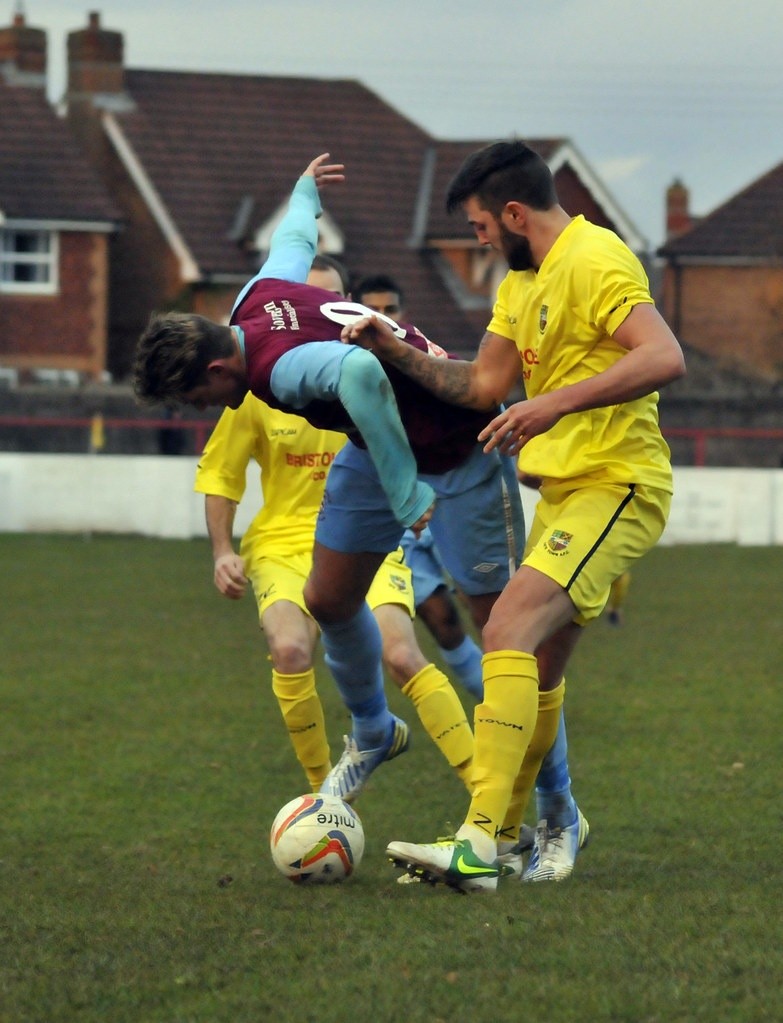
[268,792,366,887]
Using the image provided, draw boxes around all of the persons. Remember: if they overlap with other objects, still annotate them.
[360,273,495,705]
[194,243,547,861]
[130,151,593,883]
[340,142,686,891]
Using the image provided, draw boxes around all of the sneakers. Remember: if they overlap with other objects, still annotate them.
[386,840,498,896]
[397,838,523,885]
[512,822,539,855]
[521,799,589,884]
[319,709,408,799]
